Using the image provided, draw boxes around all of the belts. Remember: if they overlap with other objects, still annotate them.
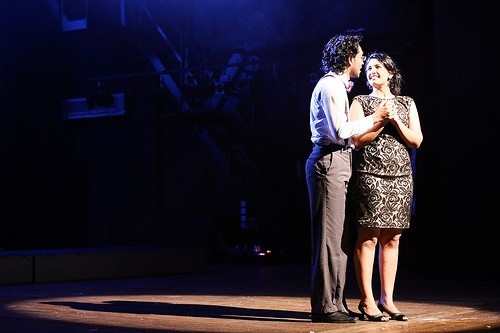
[315,143,352,151]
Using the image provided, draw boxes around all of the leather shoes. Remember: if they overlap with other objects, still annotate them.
[338,309,367,321]
[311,311,357,323]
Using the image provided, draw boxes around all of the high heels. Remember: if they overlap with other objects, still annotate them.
[377,300,408,321]
[358,300,389,322]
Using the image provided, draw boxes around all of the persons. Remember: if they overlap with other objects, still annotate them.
[305,35,388,323]
[345,52,423,322]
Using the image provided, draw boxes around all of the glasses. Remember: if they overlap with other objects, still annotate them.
[354,55,367,61]
